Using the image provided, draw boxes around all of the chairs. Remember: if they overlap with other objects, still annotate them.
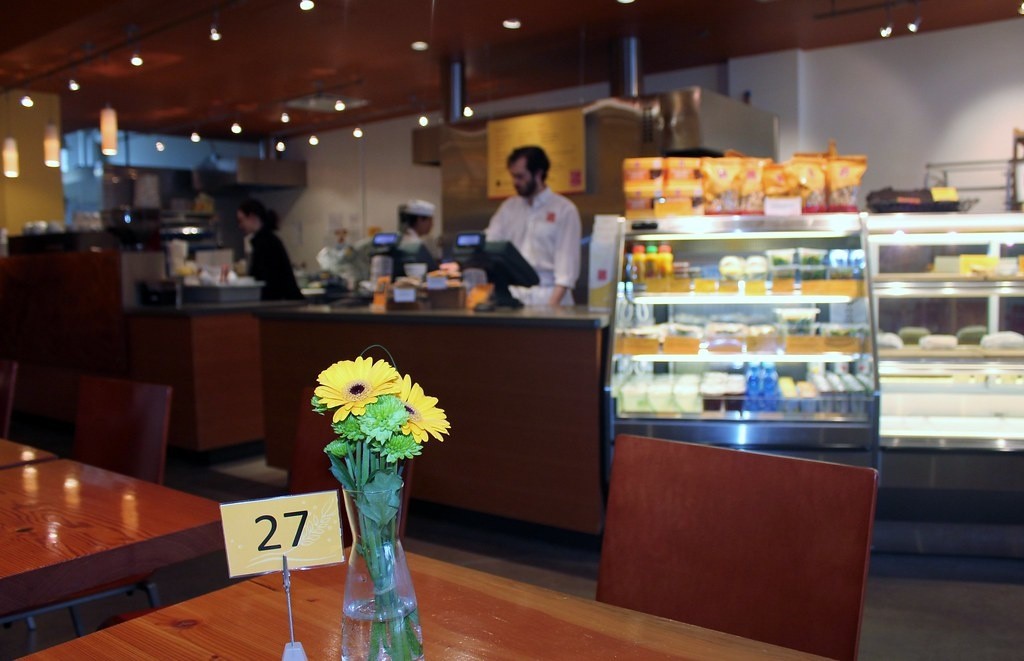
[292,387,412,547]
[596,433,878,661]
[0,361,171,638]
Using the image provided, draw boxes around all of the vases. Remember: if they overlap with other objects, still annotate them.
[341,481,424,661]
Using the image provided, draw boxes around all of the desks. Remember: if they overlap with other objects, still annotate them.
[12,545,835,661]
[0,437,226,625]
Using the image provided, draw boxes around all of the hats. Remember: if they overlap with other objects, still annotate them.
[400,199,436,218]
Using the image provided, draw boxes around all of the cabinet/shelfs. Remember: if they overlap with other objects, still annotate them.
[602,212,1024,560]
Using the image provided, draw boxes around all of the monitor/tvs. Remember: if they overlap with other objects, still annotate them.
[369,244,439,284]
[451,241,541,288]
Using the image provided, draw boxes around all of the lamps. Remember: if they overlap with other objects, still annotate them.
[2,134,20,178]
[44,121,60,167]
[100,104,117,155]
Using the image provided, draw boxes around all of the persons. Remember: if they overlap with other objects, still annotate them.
[390,199,439,283]
[485,146,582,307]
[236,200,305,303]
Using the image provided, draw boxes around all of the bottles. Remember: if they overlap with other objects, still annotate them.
[644,245,658,279]
[653,245,675,278]
[632,245,645,282]
[744,363,778,411]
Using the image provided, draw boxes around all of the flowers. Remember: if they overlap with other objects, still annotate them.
[310,356,451,661]
